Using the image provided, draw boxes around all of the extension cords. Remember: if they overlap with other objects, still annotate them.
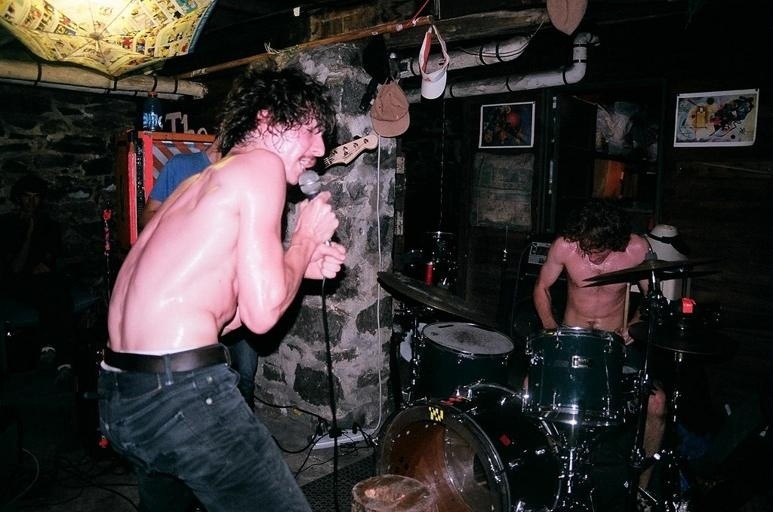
[310,428,370,450]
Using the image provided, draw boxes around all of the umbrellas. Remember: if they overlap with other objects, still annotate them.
[1,0,215,82]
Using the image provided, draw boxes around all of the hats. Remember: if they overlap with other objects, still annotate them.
[371,81,412,141]
[418,25,451,102]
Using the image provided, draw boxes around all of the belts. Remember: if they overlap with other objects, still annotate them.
[104,343,228,376]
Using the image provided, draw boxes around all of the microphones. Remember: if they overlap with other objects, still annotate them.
[96,187,114,213]
[298,168,333,246]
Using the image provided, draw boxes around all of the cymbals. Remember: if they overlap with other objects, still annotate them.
[581,257,711,282]
[628,321,718,357]
[376,271,487,324]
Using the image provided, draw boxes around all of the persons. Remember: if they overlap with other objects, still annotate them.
[92,61,350,511]
[532,204,668,492]
[137,128,224,228]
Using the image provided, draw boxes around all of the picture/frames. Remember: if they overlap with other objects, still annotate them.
[672,88,760,147]
[477,99,535,150]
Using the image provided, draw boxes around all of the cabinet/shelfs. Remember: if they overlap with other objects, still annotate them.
[540,76,667,238]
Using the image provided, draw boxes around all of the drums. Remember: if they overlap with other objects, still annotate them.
[376,385,595,511]
[352,473,433,512]
[524,327,628,428]
[409,326,514,400]
